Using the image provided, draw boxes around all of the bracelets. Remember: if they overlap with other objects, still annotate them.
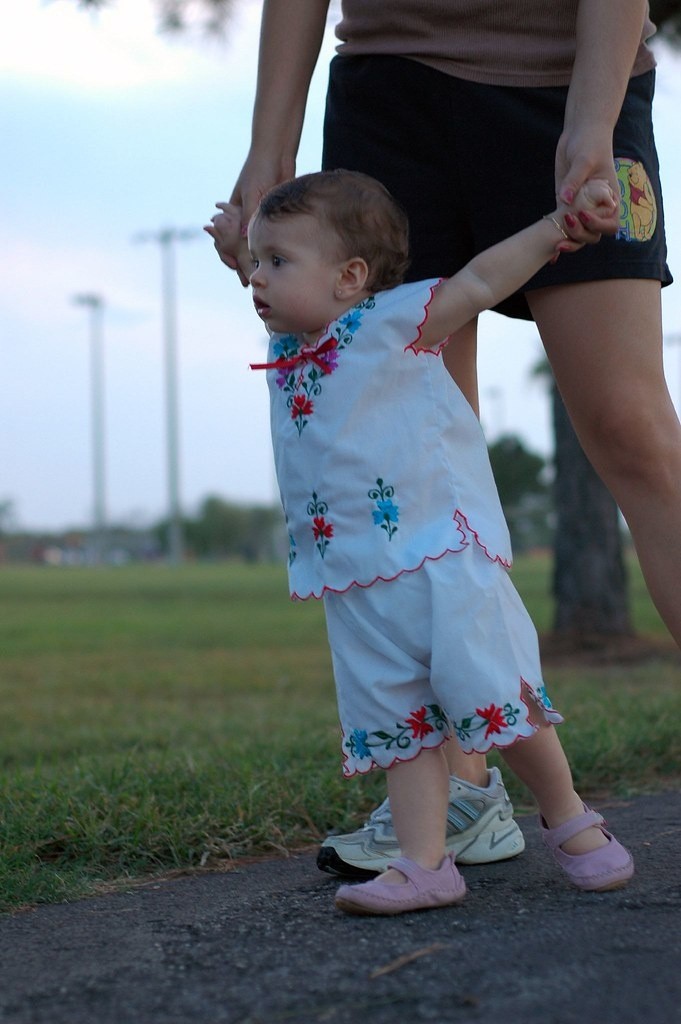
[547,214,570,240]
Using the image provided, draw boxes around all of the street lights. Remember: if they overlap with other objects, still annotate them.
[75,294,108,569]
[131,230,199,567]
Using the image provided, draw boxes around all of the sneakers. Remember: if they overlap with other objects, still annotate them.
[318,766,526,877]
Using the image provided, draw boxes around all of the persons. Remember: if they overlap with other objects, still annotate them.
[213,0,681,884]
[202,178,636,915]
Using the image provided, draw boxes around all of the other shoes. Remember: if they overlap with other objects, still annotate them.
[334,858,466,912]
[538,804,635,892]
